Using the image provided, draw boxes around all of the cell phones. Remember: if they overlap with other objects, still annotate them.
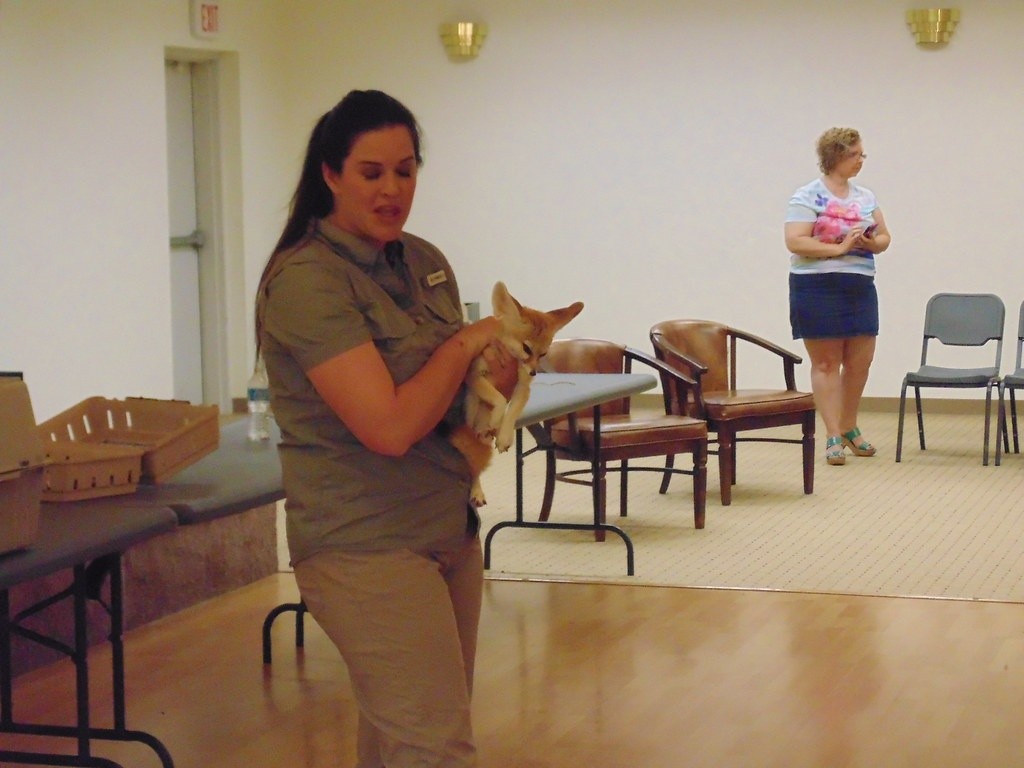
[863,223,879,238]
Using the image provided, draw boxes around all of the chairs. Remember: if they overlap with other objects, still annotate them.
[524,339,707,542]
[895,293,1009,465]
[650,318,817,506]
[995,301,1024,465]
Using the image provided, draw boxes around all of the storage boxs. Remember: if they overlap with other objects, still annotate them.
[0,371,223,556]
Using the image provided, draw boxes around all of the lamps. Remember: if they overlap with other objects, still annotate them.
[438,20,487,56]
[908,7,957,46]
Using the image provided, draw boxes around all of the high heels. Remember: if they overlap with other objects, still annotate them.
[841,427,875,456]
[826,436,845,465]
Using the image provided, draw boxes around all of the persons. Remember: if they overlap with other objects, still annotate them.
[784,128,891,465]
[254,89,520,768]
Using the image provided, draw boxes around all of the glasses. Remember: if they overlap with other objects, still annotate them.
[851,150,867,160]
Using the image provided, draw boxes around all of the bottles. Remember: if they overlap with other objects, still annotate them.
[246,362,272,442]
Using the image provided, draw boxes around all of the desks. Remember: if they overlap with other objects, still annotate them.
[0,414,307,768]
[0,494,180,768]
[264,373,658,645]
